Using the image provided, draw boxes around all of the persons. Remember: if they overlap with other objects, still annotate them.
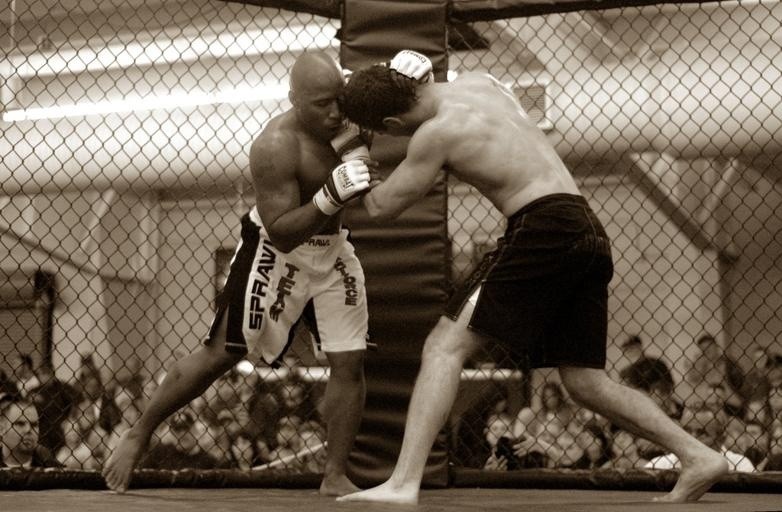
[330,65,732,506]
[449,332,782,471]
[1,351,329,472]
[99,46,436,496]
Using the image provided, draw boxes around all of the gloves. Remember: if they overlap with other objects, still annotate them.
[330,118,370,163]
